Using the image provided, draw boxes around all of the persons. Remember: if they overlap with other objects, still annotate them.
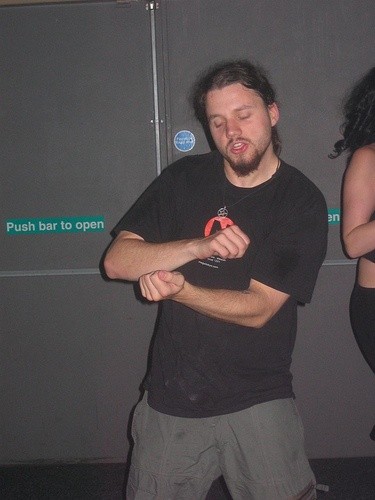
[98,57,331,500]
[327,64,374,374]
